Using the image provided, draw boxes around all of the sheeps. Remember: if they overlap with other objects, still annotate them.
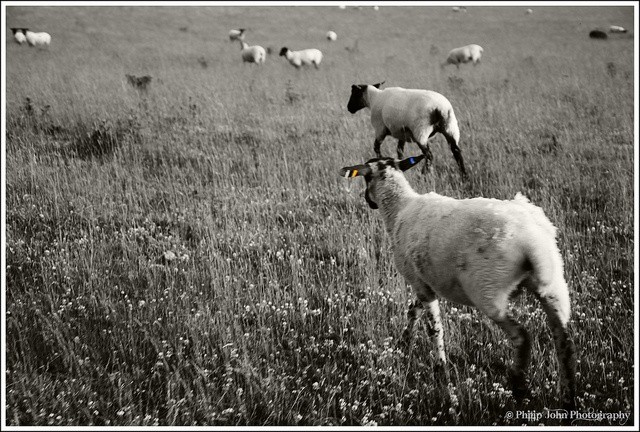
[444,45,484,69]
[346,79,468,181]
[279,46,323,70]
[240,42,265,64]
[9,27,26,44]
[229,29,244,42]
[20,27,52,46]
[339,153,579,410]
